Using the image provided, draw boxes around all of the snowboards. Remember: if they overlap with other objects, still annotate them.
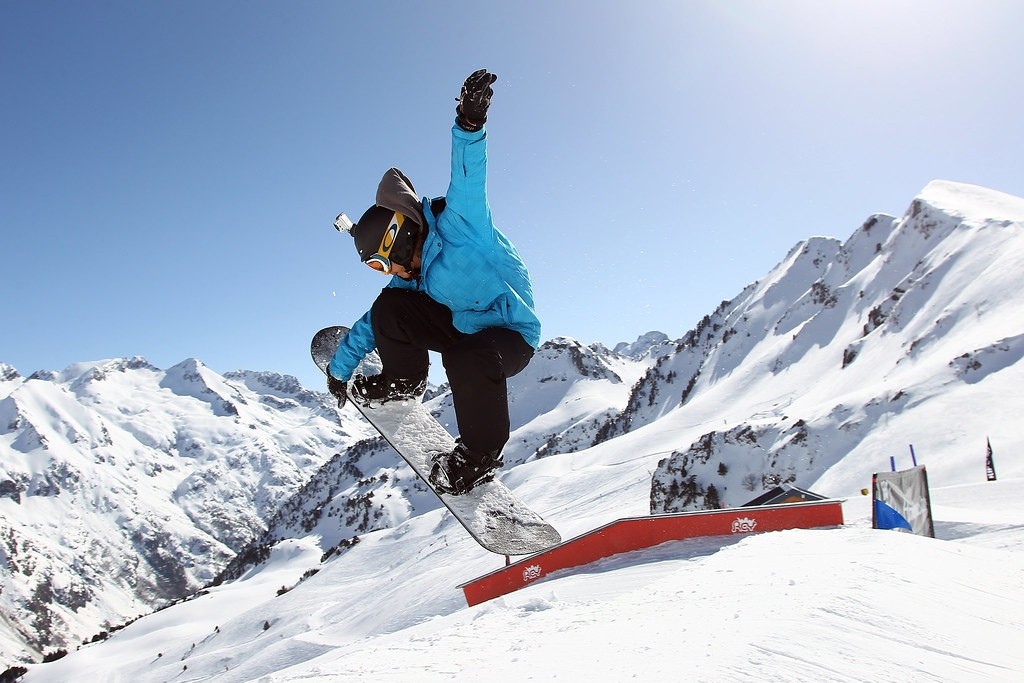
[310,324,562,556]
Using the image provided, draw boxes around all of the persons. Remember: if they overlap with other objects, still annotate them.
[326,69,541,495]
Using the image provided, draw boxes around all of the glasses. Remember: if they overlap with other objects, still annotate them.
[365,211,404,272]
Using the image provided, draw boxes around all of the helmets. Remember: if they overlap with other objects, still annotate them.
[354,204,426,283]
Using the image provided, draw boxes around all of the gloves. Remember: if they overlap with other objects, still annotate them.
[326,364,346,408]
[456,69,498,133]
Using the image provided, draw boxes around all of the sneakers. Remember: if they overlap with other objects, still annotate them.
[351,371,427,402]
[428,434,505,494]
